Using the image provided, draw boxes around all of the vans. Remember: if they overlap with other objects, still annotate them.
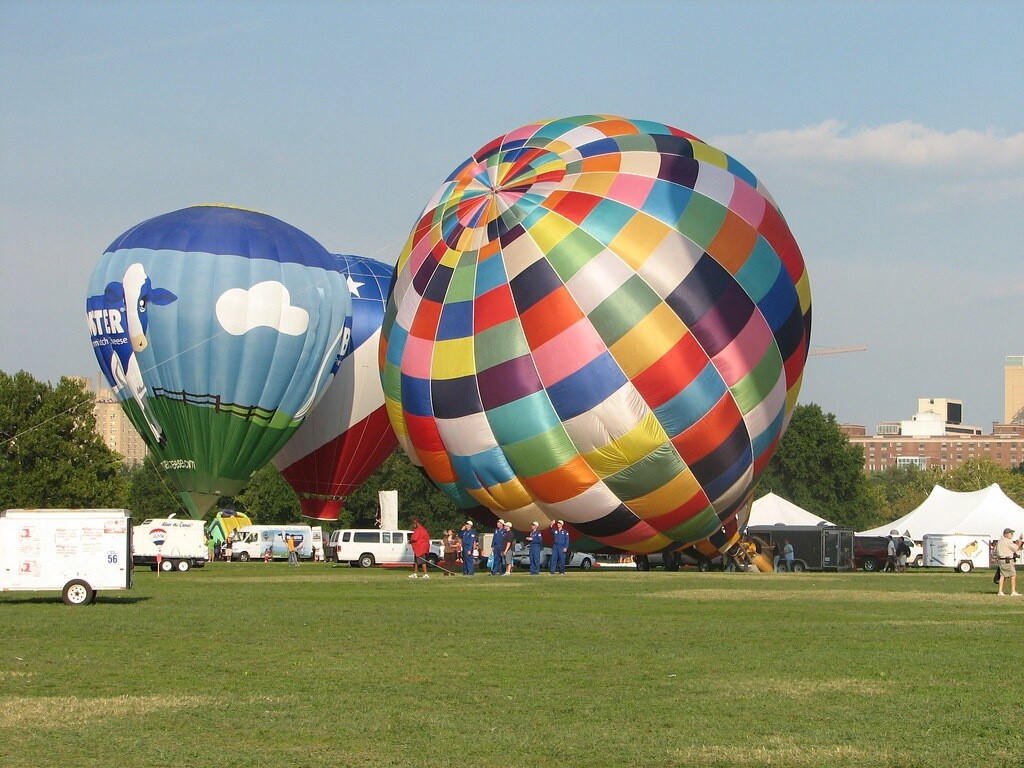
[220,525,322,562]
[326,529,441,568]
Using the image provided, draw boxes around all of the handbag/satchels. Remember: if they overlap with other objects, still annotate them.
[993,567,1006,584]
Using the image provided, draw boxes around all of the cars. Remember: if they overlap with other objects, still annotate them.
[884,535,924,568]
[513,544,595,570]
[430,539,445,558]
[852,536,889,572]
[632,552,723,572]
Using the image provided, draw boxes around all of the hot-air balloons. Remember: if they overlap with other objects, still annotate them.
[270,255,400,521]
[379,115,777,574]
[86,204,352,519]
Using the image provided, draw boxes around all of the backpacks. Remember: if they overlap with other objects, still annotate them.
[900,545,911,557]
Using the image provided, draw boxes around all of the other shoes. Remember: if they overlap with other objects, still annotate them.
[408,573,417,578]
[420,575,429,579]
[468,572,474,575]
[998,592,1007,596]
[502,572,510,576]
[1010,592,1023,596]
[560,572,564,575]
[550,571,553,575]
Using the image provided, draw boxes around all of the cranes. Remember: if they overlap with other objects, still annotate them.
[809,345,868,356]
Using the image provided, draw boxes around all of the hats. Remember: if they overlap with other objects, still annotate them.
[558,520,564,525]
[504,522,513,528]
[532,522,539,527]
[496,518,504,525]
[466,521,472,525]
[1004,528,1015,534]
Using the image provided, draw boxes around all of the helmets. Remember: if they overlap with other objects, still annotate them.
[412,519,419,524]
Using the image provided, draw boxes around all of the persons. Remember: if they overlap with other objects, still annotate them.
[761,539,782,573]
[997,528,1023,597]
[459,520,477,576]
[490,519,522,577]
[204,536,233,563]
[883,536,909,573]
[443,528,459,576]
[781,537,794,573]
[287,534,299,567]
[525,521,542,575]
[408,519,430,578]
[549,519,570,576]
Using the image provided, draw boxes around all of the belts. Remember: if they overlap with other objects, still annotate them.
[999,558,1014,560]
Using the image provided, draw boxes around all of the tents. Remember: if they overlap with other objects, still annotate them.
[855,484,1024,565]
[744,489,836,568]
